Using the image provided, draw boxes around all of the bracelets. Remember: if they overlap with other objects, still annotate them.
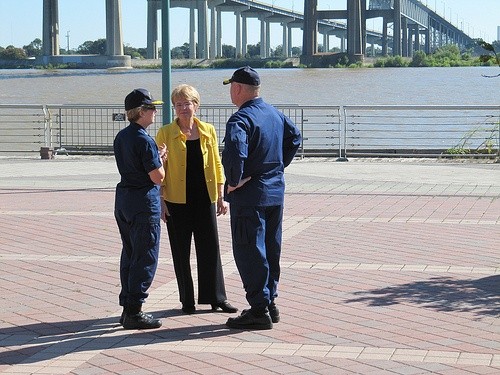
[219,197,224,199]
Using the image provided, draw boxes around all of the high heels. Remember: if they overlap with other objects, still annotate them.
[212,302,238,313]
[182,302,196,313]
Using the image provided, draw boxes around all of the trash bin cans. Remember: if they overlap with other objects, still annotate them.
[41,147,54,159]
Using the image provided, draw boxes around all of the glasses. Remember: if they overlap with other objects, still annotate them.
[142,106,155,110]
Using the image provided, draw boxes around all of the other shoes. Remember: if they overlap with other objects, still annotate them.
[119,310,154,324]
[226,307,273,330]
[123,311,162,329]
[241,304,280,323]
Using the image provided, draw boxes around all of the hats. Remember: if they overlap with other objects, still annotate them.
[125,88,164,111]
[223,65,260,87]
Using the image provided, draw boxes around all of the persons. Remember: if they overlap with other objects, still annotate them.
[221,67,302,329]
[113,89,169,331]
[153,83,240,313]
